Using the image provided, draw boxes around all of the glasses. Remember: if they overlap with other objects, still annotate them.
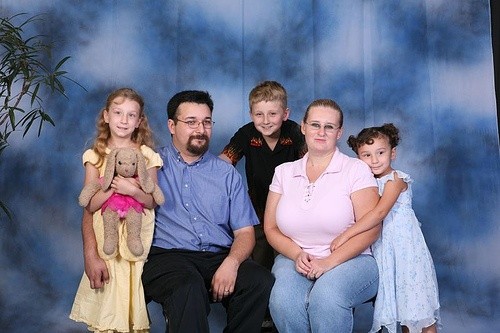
[173,117,215,129]
[305,121,340,134]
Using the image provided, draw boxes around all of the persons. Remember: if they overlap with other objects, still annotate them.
[264,98,380,333]
[216,83,307,327]
[69,88,163,333]
[82,90,276,333]
[330,123,441,333]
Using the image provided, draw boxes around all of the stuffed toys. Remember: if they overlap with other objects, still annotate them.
[79,147,166,256]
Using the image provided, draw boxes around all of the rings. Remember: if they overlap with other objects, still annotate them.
[312,272,316,276]
[224,289,229,292]
